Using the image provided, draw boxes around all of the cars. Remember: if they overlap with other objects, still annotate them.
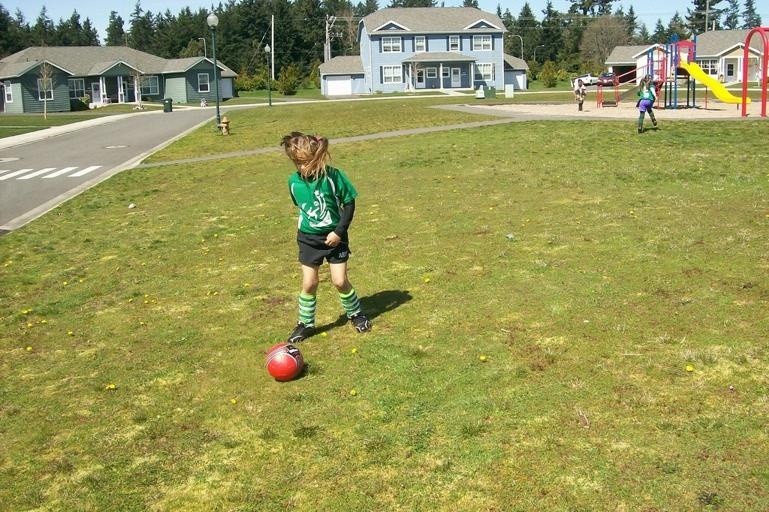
[571,73,598,89]
[598,72,620,88]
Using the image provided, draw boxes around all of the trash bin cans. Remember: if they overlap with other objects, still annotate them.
[163,99,172,113]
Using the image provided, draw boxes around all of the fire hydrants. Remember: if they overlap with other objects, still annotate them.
[217,115,233,137]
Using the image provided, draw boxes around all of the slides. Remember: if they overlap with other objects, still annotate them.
[679,61,751,104]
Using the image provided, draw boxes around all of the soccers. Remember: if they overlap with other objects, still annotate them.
[266,342,304,381]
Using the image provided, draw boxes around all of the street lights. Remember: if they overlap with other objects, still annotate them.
[207,9,222,132]
[508,34,526,60]
[198,37,209,57]
[264,42,274,108]
[533,45,545,62]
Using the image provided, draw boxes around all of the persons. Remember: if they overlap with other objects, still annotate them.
[575,79,586,111]
[280,131,371,342]
[638,75,657,132]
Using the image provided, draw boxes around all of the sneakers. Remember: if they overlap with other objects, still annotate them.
[288,321,315,343]
[347,310,371,333]
